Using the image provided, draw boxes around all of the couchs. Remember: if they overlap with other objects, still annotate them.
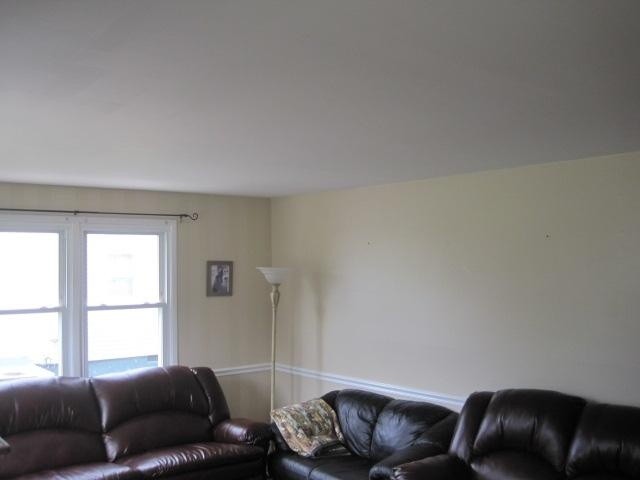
[0,365,268,480]
[268,390,459,479]
[389,389,640,480]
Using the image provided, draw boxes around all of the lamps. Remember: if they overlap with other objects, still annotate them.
[256,266,289,421]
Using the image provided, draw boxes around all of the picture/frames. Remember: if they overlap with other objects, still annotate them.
[206,260,234,297]
